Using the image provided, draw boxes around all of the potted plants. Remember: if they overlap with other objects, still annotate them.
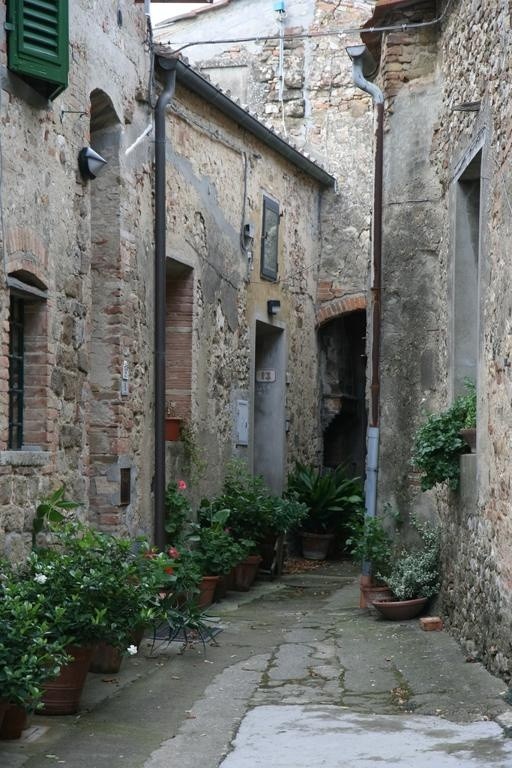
[165,416,210,483]
[458,377,476,453]
[157,475,288,611]
[284,456,363,561]
[1,523,154,741]
[341,501,442,618]
